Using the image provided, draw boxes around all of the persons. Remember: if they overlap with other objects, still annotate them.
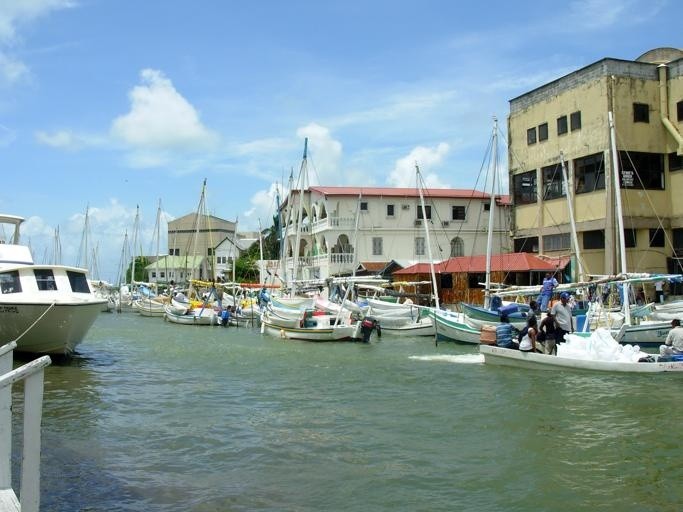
[496,272,574,356]
[659,319,683,357]
[654,281,665,303]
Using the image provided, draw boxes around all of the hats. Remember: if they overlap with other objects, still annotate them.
[560,293,571,299]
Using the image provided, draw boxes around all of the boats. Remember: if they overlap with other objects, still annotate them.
[0,212,106,357]
